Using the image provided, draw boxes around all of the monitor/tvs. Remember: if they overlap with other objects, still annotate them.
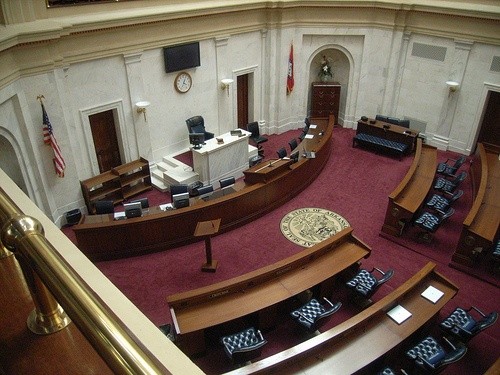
[124,202,142,219]
[189,133,205,149]
[291,150,300,164]
[163,42,201,72]
[172,191,190,208]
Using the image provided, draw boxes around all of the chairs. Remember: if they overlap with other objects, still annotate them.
[197,184,213,194]
[276,146,287,158]
[95,201,115,214]
[376,114,410,128]
[414,208,455,242]
[219,176,236,188]
[434,172,466,194]
[303,127,308,134]
[441,306,497,344]
[121,198,149,209]
[427,190,464,213]
[406,336,469,371]
[248,122,268,153]
[304,118,310,128]
[290,299,342,339]
[221,328,268,366]
[169,184,188,203]
[380,368,408,375]
[185,116,214,140]
[287,138,297,151]
[343,266,394,305]
[297,132,306,142]
[437,155,464,177]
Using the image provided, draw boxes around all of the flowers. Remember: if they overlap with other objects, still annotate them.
[319,55,334,80]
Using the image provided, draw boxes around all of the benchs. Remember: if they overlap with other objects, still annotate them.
[352,134,406,162]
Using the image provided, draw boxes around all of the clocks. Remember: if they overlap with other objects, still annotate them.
[135,101,150,121]
[174,71,192,93]
[221,79,234,97]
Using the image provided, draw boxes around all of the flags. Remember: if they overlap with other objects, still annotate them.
[287,47,295,95]
[42,106,66,178]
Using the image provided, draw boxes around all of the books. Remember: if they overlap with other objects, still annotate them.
[388,304,412,324]
[422,286,444,303]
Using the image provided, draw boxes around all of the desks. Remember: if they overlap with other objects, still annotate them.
[72,115,500,375]
[190,127,252,182]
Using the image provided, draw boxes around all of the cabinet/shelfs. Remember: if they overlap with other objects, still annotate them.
[312,83,340,128]
[80,156,152,215]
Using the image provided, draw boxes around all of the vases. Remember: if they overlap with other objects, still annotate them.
[321,74,329,83]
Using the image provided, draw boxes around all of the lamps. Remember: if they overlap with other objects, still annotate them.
[447,81,459,93]
